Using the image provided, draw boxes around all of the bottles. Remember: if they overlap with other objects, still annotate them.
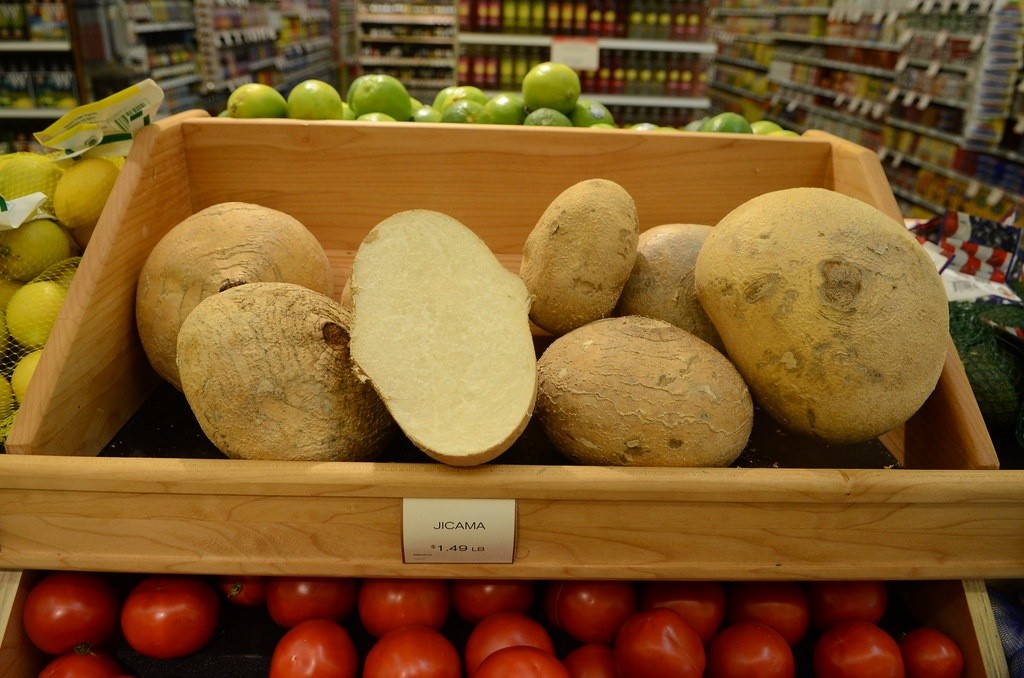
[605,104,695,130]
[457,0,700,42]
[457,46,707,97]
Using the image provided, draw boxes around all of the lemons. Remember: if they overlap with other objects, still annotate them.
[0,151,127,442]
[212,63,802,138]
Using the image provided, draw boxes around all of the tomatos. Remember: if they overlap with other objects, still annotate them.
[19,560,968,678]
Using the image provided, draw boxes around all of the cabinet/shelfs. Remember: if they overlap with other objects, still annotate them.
[0,0,1023,261]
